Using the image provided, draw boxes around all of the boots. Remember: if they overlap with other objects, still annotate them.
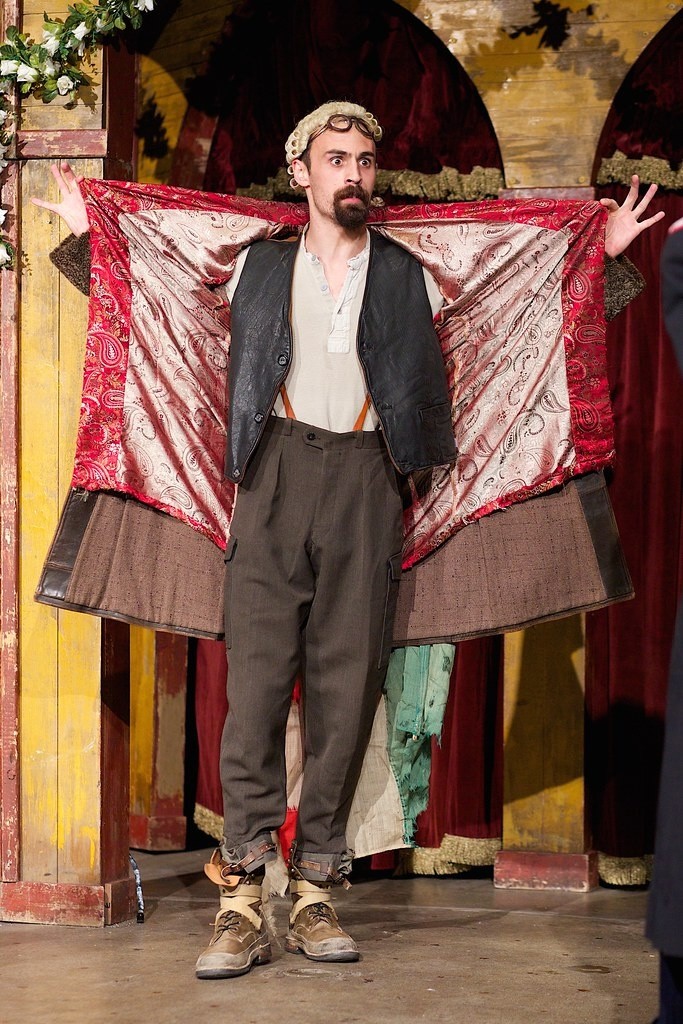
[284,843,359,962]
[194,847,270,978]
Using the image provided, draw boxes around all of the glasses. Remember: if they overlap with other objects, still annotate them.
[307,113,374,140]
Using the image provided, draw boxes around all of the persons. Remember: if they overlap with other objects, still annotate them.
[33,100,666,981]
